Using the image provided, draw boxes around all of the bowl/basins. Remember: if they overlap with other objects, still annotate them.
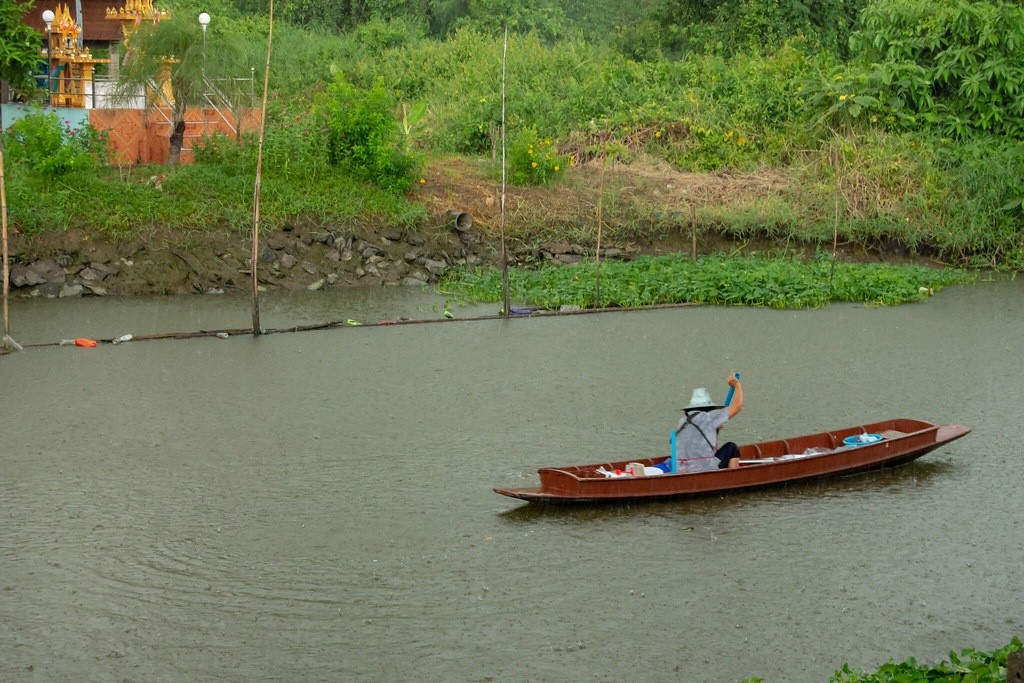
[843,434,883,446]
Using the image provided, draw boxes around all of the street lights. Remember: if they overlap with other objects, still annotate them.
[42,9,55,108]
[199,12,210,106]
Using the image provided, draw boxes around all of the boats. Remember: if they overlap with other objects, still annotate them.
[493,418,972,503]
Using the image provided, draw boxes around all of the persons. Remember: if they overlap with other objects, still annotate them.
[670,373,743,473]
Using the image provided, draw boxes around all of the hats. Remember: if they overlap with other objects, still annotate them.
[680,388,726,410]
